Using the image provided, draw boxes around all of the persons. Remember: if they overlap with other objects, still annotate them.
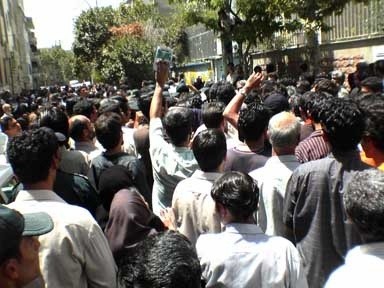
[0,45,384,288]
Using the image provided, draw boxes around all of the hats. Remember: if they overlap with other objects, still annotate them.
[0,204,53,250]
[265,94,290,113]
[99,98,119,112]
[163,107,188,127]
